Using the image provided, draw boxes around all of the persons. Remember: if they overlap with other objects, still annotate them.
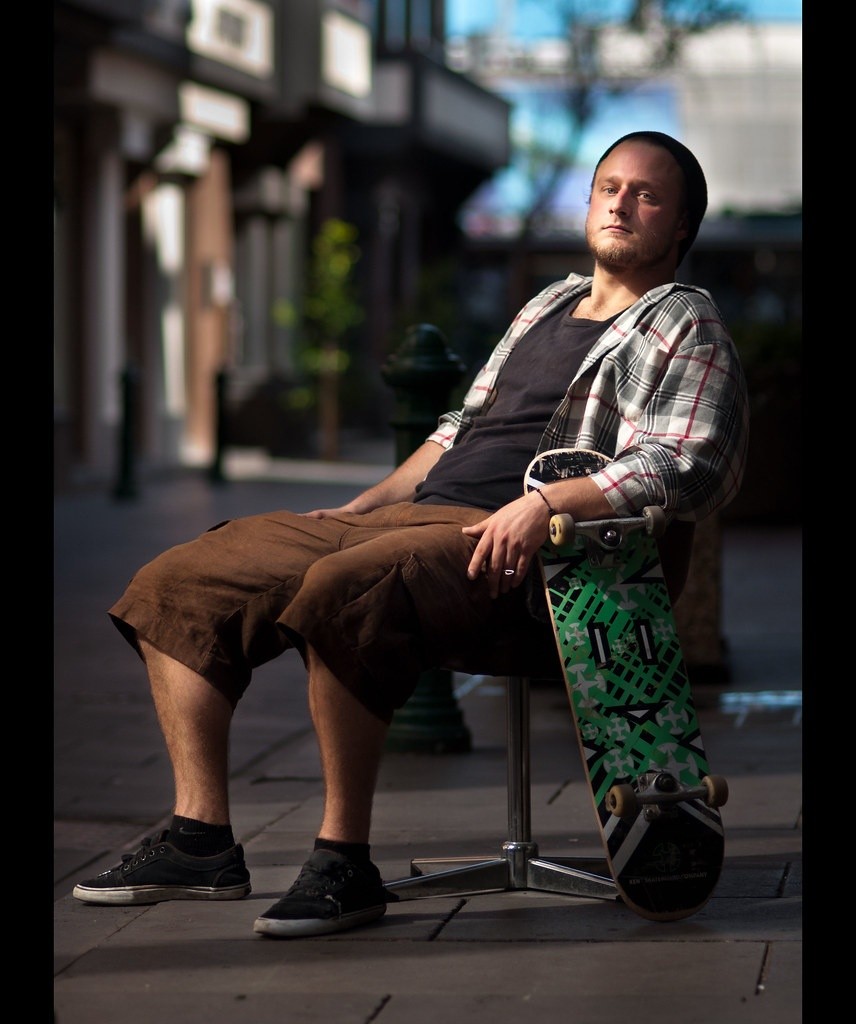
[72,131,750,938]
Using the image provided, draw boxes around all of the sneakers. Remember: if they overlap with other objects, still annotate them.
[72,829,252,905]
[253,849,387,937]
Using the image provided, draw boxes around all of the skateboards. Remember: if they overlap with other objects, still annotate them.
[520,445,733,925]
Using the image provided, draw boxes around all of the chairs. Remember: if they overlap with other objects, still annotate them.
[381,519,696,908]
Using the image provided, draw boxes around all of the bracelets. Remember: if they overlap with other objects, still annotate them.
[535,488,556,515]
